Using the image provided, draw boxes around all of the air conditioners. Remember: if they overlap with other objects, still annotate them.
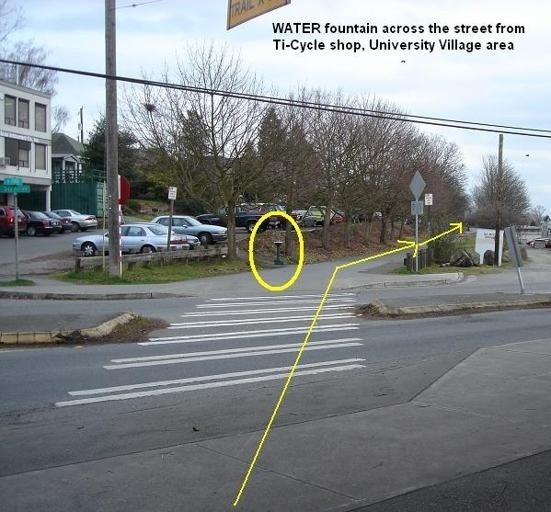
[0,157,11,166]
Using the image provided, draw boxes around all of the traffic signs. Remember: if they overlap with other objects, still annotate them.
[0,178,31,194]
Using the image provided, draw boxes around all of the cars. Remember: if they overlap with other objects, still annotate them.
[1,206,97,238]
[195,203,345,234]
[73,216,228,257]
